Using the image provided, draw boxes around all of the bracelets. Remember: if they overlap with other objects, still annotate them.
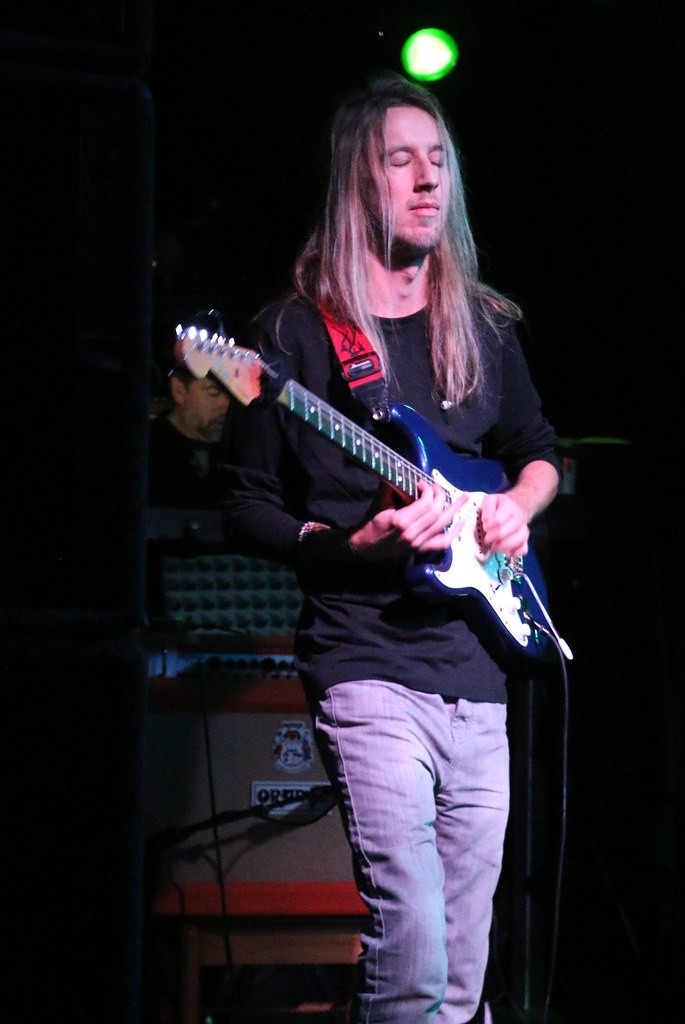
[299,521,330,542]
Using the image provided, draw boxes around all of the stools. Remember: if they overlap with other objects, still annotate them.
[148,880,370,1024]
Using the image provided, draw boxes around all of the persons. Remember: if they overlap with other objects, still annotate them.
[205,75,564,1024]
[148,365,230,507]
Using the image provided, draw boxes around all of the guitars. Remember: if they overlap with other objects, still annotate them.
[172,321,555,681]
